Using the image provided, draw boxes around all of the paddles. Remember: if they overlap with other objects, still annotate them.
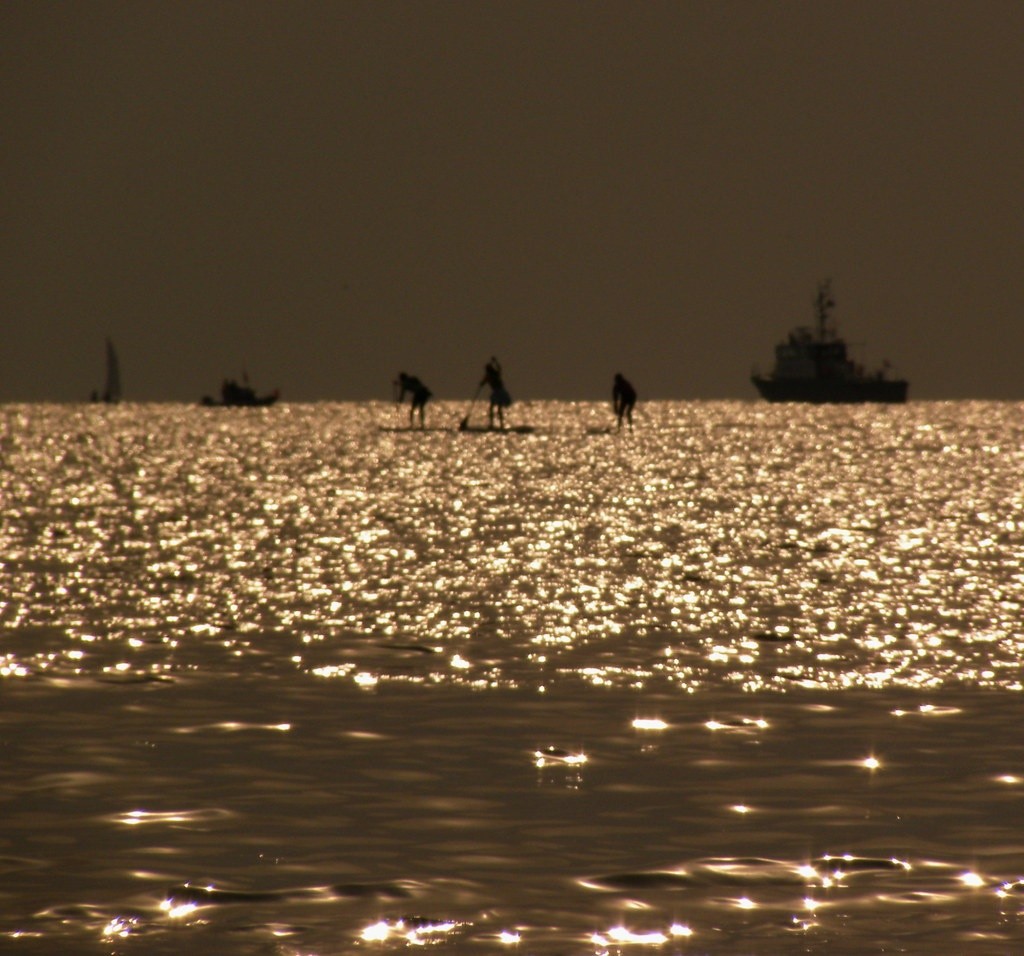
[391,383,455,428]
[459,377,492,430]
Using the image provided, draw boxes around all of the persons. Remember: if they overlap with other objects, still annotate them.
[395,371,433,431]
[613,372,637,432]
[478,355,512,430]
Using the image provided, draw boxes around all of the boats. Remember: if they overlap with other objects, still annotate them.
[201,380,280,409]
[749,278,914,406]
